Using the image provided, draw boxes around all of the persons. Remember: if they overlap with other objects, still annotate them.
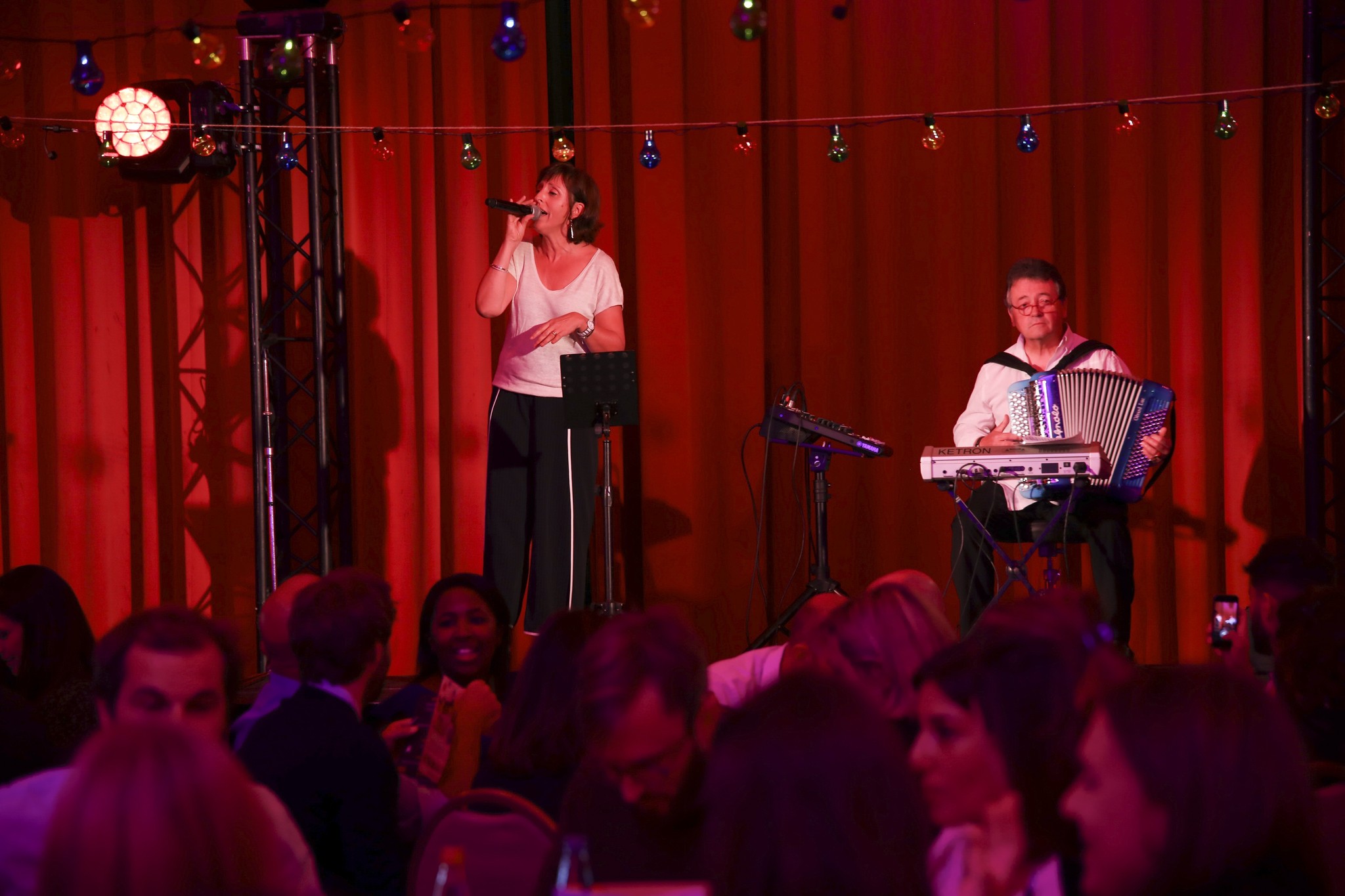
[2,536,1345,896]
[480,162,625,638]
[946,258,1172,664]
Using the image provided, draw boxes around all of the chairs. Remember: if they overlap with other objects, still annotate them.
[404,790,565,896]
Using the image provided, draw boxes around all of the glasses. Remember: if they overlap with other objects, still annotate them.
[594,722,688,782]
[1011,297,1059,316]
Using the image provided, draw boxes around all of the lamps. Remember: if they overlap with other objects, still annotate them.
[94,78,244,185]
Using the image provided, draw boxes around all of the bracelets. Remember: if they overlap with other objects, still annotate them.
[581,329,594,344]
[490,264,509,273]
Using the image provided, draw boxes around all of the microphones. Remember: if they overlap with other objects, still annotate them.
[485,198,541,221]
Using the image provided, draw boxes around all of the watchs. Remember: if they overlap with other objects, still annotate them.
[577,320,595,337]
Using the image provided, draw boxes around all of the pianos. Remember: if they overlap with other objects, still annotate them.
[921,442,1102,629]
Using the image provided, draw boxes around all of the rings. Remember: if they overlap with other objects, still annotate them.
[549,331,557,336]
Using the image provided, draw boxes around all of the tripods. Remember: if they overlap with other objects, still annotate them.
[732,449,851,654]
